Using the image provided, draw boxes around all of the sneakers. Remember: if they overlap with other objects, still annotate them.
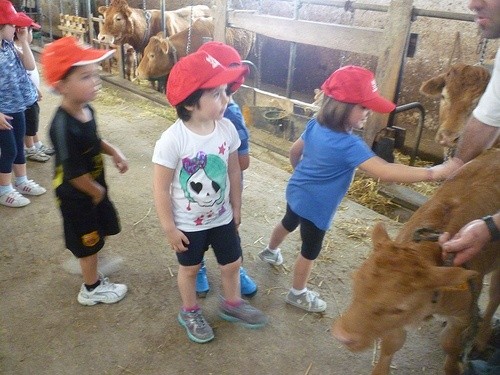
[196,259,210,293]
[177,310,215,343]
[24,150,50,162]
[0,189,31,207]
[78,271,128,306]
[38,145,56,155]
[257,248,284,265]
[240,265,257,295]
[287,290,327,312]
[216,294,266,328]
[14,180,47,195]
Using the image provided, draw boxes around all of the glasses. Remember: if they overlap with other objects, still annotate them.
[8,24,17,28]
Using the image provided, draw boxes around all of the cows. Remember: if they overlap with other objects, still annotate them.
[94,0,213,93]
[419,57,496,158]
[328,149,500,375]
[132,15,259,81]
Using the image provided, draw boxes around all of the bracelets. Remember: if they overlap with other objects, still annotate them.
[482,216,500,240]
[427,168,433,182]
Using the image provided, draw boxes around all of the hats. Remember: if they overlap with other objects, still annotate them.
[16,12,41,29]
[181,42,246,91]
[167,50,249,107]
[43,37,117,88]
[322,66,396,113]
[0,0,33,26]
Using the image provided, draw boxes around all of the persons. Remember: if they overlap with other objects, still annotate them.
[154,50,268,343]
[42,35,128,305]
[196,42,258,297]
[13,13,55,162]
[258,65,463,312]
[0,0,46,208]
[439,0,500,375]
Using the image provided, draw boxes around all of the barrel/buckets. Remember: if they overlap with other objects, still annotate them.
[262,110,288,133]
